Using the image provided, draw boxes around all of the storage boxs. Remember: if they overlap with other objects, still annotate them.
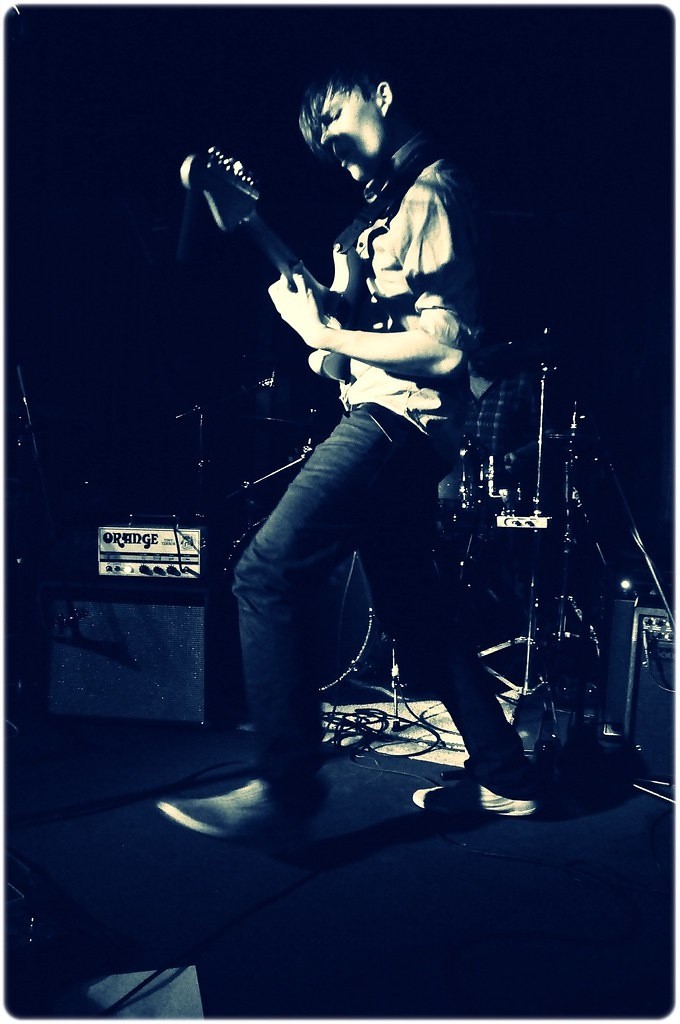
[39,581,226,732]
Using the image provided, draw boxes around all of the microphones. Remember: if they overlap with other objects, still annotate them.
[269,369,281,418]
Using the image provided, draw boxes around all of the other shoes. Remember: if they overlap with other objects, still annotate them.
[412,781,537,816]
[156,778,320,842]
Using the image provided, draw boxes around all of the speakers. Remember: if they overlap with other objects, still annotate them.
[37,587,233,734]
[621,607,676,800]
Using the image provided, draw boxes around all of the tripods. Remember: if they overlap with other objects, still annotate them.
[476,327,607,759]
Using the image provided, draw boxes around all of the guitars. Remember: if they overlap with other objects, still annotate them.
[178,142,372,383]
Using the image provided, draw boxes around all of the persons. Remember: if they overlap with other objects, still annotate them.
[157,61,538,838]
